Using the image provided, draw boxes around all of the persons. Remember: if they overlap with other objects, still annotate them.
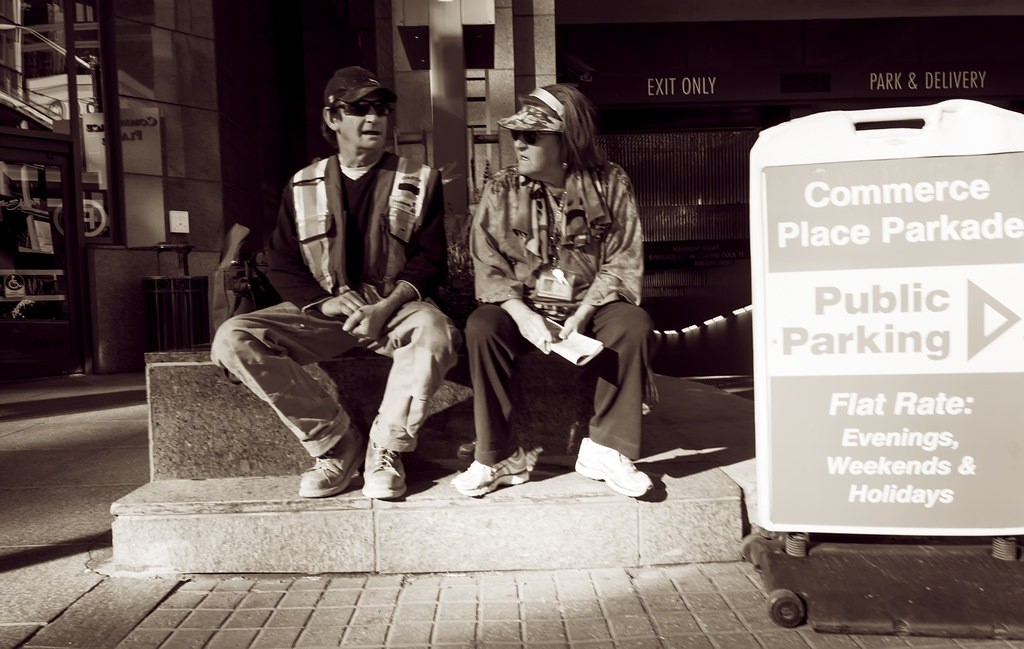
[450,85,654,497]
[210,66,461,500]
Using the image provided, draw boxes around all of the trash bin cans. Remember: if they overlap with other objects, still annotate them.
[140,242,213,353]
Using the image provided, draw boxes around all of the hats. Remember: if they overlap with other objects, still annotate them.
[326,66,400,108]
[496,102,567,133]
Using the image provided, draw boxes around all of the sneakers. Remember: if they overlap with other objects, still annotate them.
[455,446,530,496]
[575,438,653,497]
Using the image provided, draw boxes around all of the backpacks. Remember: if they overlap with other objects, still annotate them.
[228,257,283,310]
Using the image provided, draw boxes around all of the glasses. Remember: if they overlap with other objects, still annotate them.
[334,101,391,117]
[509,129,537,144]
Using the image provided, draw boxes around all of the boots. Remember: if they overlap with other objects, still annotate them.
[362,435,408,498]
[298,429,365,498]
